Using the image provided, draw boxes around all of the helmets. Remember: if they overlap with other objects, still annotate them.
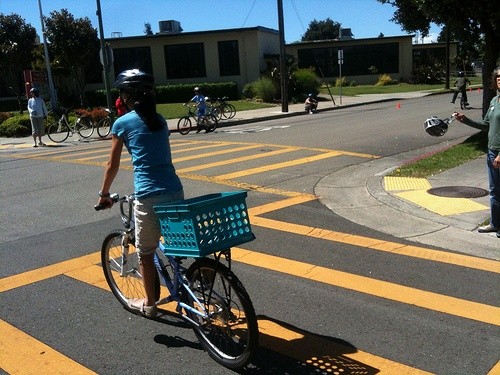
[111,68,154,92]
[30,89,37,92]
[459,71,464,76]
[194,87,199,91]
[424,116,447,137]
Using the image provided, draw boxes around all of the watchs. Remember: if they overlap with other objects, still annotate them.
[98,190,110,198]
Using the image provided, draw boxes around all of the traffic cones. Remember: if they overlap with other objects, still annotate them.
[469,88,472,92]
[477,88,480,92]
[396,104,400,109]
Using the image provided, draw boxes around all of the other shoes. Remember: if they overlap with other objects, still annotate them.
[198,267,215,290]
[33,141,37,147]
[127,299,158,317]
[205,130,211,133]
[450,101,455,104]
[465,103,469,106]
[196,129,200,134]
[478,224,500,238]
[38,142,45,145]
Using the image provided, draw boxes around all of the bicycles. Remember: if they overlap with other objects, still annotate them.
[94,192,260,370]
[96,106,120,138]
[47,110,94,143]
[177,96,236,135]
[455,84,469,109]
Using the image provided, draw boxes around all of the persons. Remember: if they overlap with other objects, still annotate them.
[453,67,500,237]
[97,68,183,317]
[183,86,212,134]
[304,94,319,114]
[450,71,471,106]
[27,88,49,146]
